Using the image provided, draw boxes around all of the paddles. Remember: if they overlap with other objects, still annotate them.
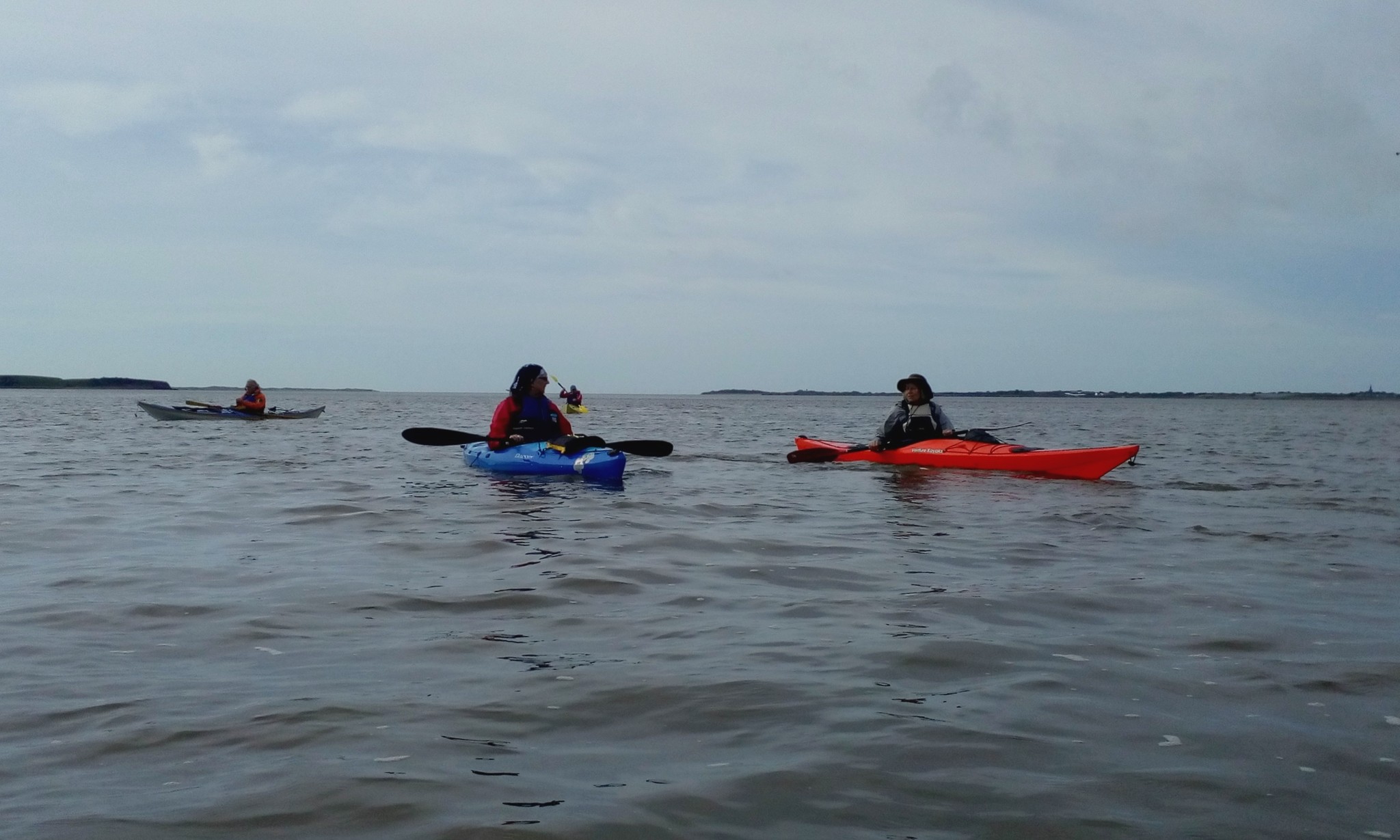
[402,427,673,457]
[551,376,587,413]
[787,422,1032,463]
[186,400,265,417]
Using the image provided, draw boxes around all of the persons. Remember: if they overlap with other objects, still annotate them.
[560,385,582,406]
[488,363,573,449]
[868,373,956,451]
[236,380,266,413]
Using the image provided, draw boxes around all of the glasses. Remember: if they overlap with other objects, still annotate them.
[539,377,548,381]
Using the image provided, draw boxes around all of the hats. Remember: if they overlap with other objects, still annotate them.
[571,386,576,390]
[246,379,258,388]
[897,374,934,399]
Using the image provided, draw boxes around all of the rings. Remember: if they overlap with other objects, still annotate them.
[517,435,519,437]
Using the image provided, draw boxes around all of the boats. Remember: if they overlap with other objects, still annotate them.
[794,435,1140,481]
[461,441,627,478]
[131,398,327,421]
[560,403,589,414]
